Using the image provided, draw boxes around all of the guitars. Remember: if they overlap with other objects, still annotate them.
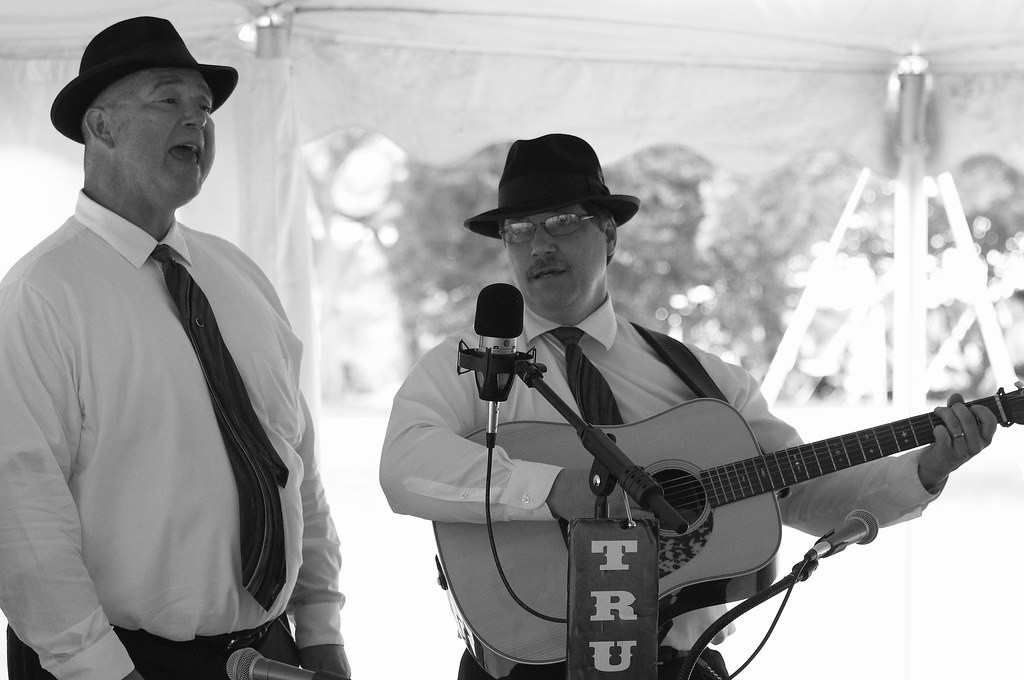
[431,384,1024,679]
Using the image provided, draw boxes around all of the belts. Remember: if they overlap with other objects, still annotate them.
[186,617,283,652]
[655,645,692,664]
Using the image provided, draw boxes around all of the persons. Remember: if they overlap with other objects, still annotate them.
[1,17,351,680]
[379,134,997,680]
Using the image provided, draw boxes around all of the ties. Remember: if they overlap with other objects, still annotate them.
[150,243,290,612]
[543,326,673,649]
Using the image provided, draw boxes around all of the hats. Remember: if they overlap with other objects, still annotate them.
[462,133,641,241]
[51,15,238,146]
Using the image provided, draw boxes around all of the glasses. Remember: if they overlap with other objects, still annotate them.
[497,213,609,243]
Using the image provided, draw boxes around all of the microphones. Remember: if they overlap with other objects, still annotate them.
[803,510,880,561]
[227,648,352,680]
[474,282,525,447]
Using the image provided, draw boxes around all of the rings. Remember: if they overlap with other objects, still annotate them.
[952,433,964,438]
